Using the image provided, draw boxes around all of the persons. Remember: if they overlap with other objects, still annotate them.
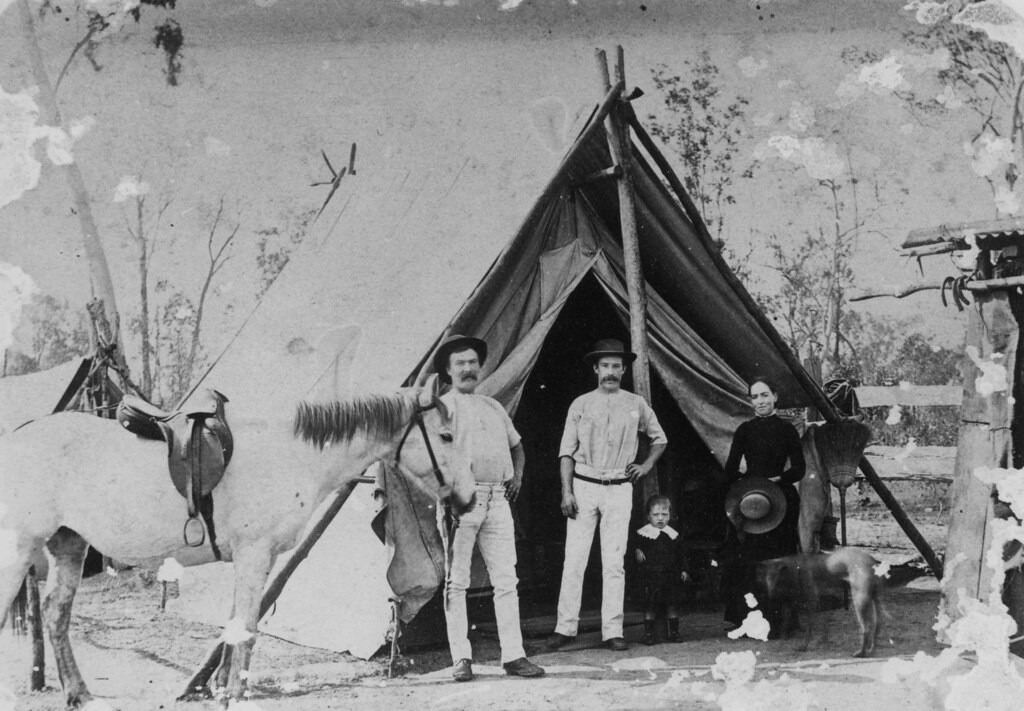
[434,335,545,681]
[721,377,807,637]
[546,338,668,650]
[630,495,688,644]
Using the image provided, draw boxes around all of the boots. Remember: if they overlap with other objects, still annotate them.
[669,618,683,643]
[645,617,655,645]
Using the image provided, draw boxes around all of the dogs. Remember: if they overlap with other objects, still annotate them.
[750,547,896,658]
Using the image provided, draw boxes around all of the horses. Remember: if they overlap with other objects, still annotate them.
[0,372,477,711]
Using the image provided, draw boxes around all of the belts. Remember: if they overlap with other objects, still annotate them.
[476,484,506,492]
[574,470,631,485]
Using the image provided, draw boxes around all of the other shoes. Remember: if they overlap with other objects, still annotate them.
[725,624,741,631]
[768,625,778,640]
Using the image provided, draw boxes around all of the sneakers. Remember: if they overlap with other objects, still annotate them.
[454,657,473,680]
[547,630,575,647]
[504,656,545,675]
[608,636,629,649]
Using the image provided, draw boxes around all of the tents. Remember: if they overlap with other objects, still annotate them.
[1,100,835,658]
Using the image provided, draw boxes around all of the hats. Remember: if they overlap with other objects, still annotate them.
[584,339,637,366]
[725,476,787,534]
[433,335,487,384]
